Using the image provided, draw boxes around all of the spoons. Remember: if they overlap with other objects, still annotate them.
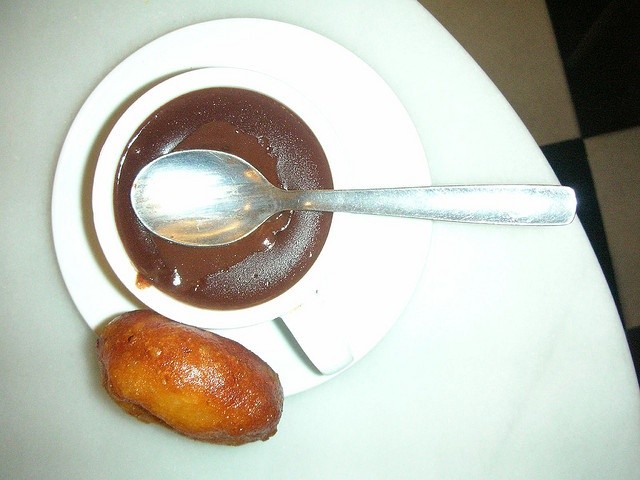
[130,148,578,247]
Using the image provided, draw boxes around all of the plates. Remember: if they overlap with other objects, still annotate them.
[90,65,343,330]
[50,16,432,397]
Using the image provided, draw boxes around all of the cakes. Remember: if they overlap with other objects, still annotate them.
[96,313,283,444]
